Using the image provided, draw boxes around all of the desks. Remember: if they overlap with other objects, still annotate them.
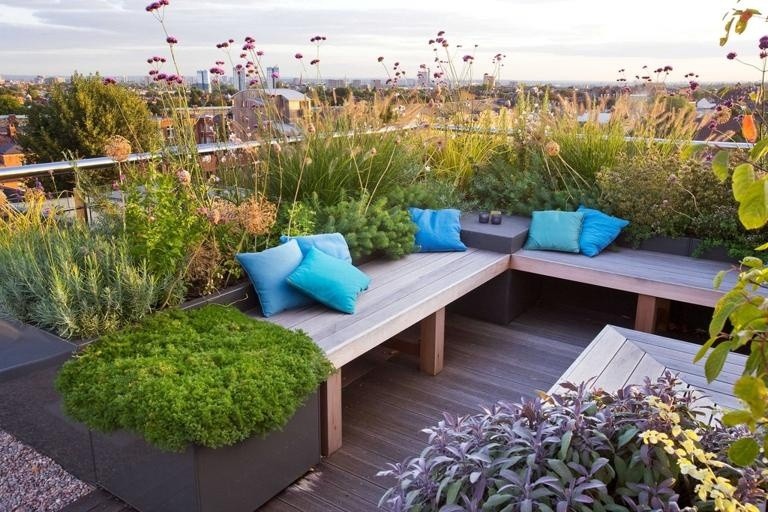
[549,321,758,441]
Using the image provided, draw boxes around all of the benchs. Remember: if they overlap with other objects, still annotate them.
[242,245,511,459]
[511,244,768,336]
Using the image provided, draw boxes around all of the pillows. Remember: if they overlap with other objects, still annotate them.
[575,205,629,257]
[234,237,316,318]
[406,207,468,252]
[523,210,584,254]
[280,232,353,265]
[285,245,370,315]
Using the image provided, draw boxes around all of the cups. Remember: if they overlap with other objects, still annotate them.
[479,212,489,223]
[491,214,502,224]
